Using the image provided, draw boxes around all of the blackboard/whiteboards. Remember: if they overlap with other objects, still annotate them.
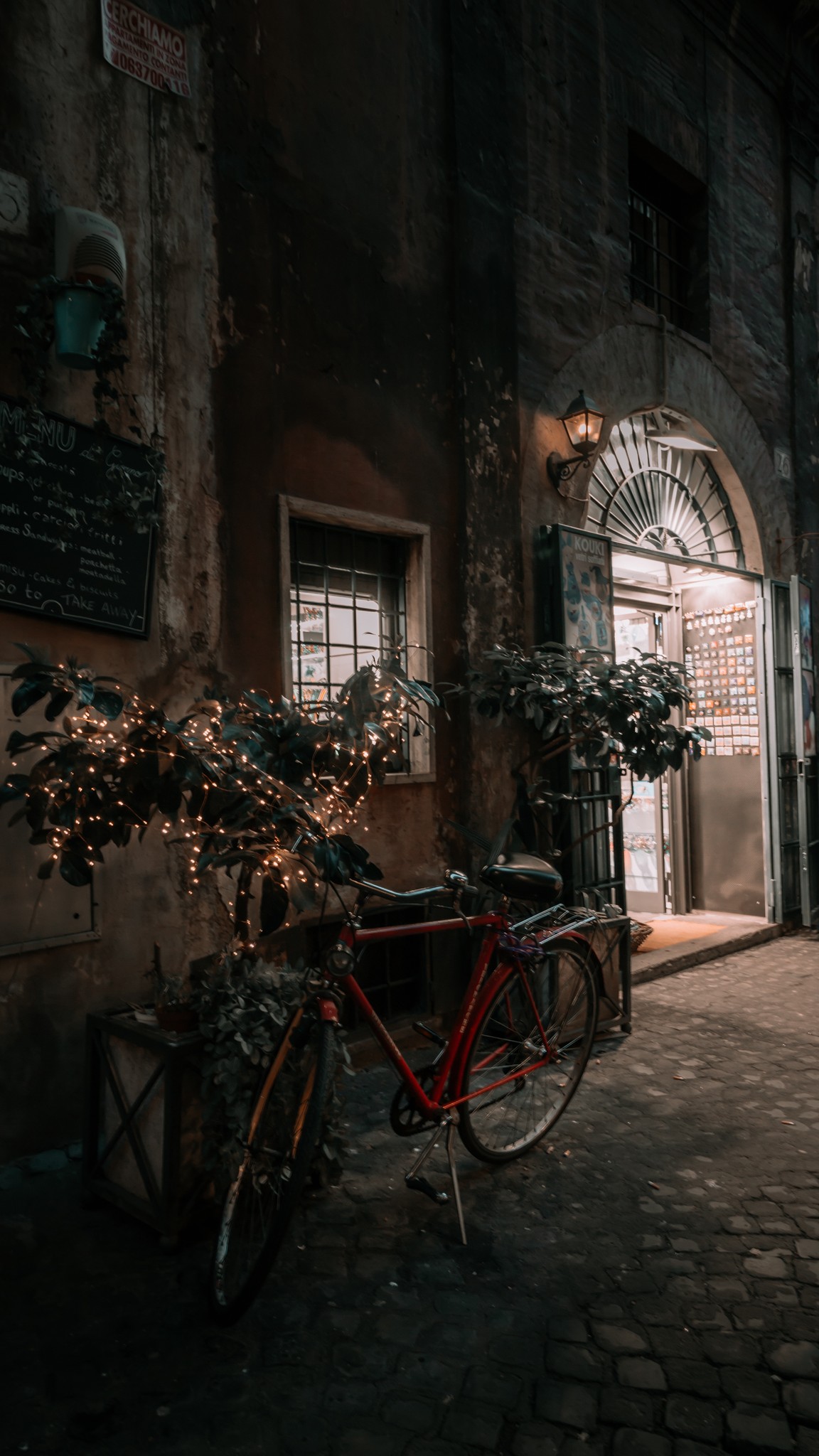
[0,392,162,641]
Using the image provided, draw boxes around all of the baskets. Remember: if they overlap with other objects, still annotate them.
[628,920,654,952]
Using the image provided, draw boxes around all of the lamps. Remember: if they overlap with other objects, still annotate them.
[547,388,604,499]
[643,409,719,452]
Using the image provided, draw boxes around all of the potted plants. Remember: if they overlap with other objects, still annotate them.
[1,598,449,1255]
[426,636,714,1038]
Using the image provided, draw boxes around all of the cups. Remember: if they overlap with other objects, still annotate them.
[604,904,623,918]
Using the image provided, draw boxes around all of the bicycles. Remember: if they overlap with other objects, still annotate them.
[207,820,607,1330]
